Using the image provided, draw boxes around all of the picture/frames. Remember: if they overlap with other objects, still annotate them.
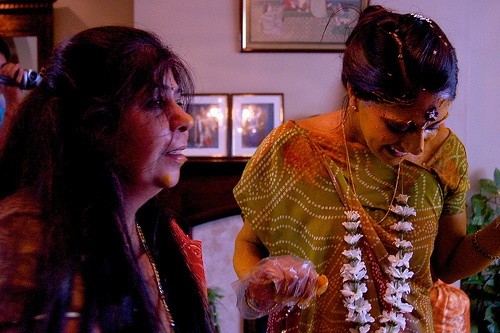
[238,0,372,54]
[230,92,285,160]
[172,92,230,159]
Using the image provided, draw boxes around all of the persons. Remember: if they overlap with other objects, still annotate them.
[234,3,499,333]
[0,25,222,333]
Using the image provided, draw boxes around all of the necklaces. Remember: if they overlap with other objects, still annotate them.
[340,109,400,224]
[135,220,178,332]
[339,156,418,333]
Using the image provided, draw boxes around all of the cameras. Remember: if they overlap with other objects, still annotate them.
[5,64,42,88]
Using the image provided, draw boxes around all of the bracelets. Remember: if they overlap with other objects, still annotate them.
[244,288,278,314]
[471,229,500,260]
